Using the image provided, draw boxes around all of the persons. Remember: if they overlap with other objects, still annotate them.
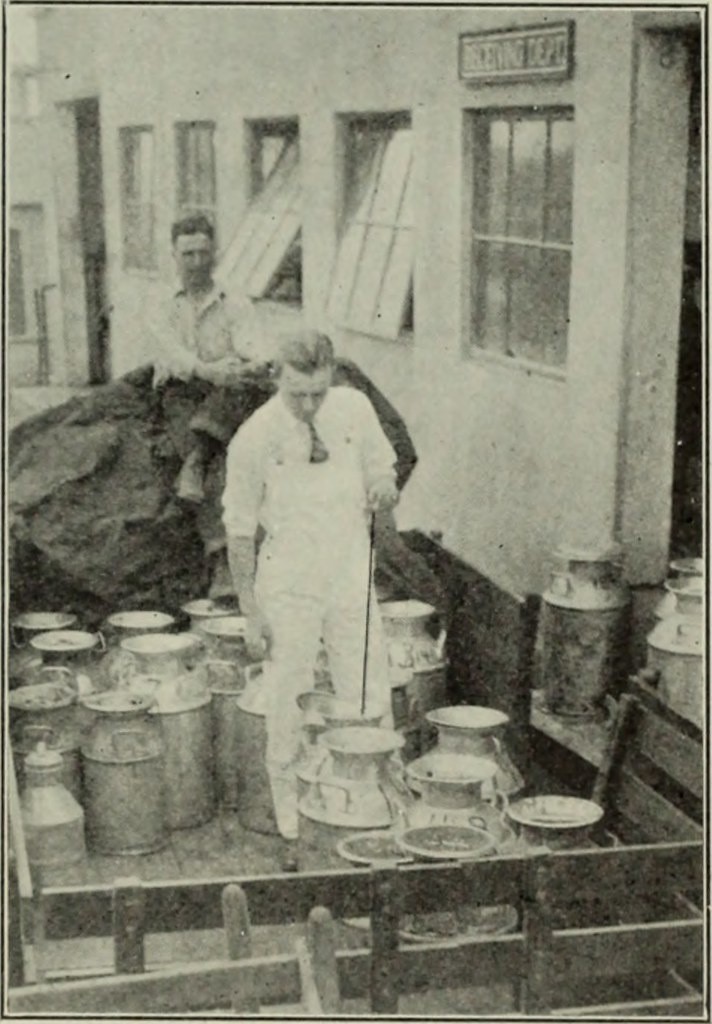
[220,330,409,844]
[150,214,275,610]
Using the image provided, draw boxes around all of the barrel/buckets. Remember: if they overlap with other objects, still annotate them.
[546,545,633,723]
[641,554,703,726]
[296,600,605,865]
[10,598,282,888]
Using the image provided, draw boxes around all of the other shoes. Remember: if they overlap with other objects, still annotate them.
[167,447,207,504]
[206,550,238,605]
